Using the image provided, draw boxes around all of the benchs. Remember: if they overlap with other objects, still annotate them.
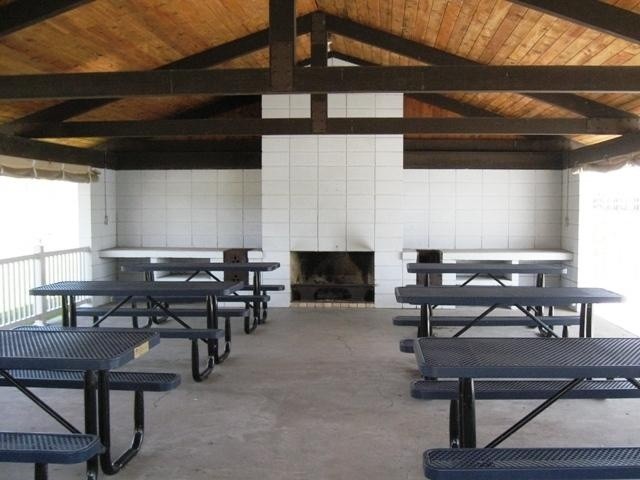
[0,284,285,480]
[393,284,640,479]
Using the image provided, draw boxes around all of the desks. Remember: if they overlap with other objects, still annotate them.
[120,262,280,334]
[407,264,568,337]
